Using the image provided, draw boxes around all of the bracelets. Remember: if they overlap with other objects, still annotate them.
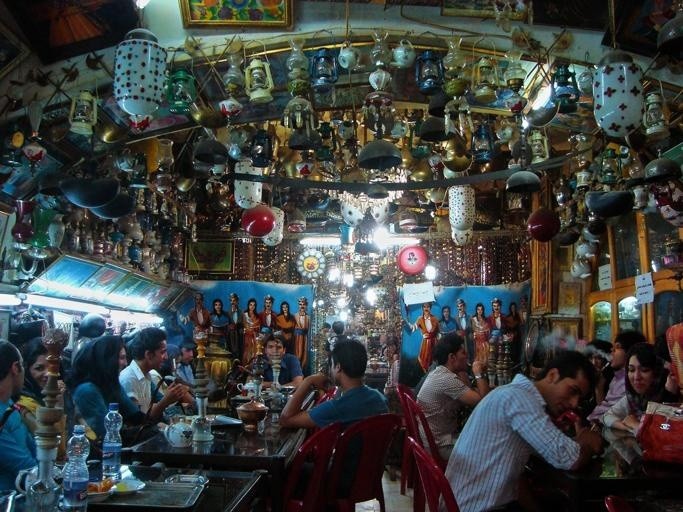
[304,378,313,391]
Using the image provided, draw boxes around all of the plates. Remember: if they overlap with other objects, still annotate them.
[88,482,117,501]
[113,479,146,492]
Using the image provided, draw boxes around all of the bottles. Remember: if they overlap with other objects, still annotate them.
[102,403,123,480]
[62,424,90,512]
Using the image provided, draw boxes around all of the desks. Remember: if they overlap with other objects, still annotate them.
[522,418,653,512]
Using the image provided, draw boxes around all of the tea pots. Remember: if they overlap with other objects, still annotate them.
[575,242,596,258]
[389,38,417,68]
[338,40,365,71]
[236,379,254,391]
[571,260,592,278]
[14,462,70,495]
[164,418,193,447]
[582,228,600,243]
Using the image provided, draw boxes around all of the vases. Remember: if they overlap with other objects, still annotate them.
[10,187,193,287]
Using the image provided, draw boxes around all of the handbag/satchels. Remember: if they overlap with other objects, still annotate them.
[636,401,682,461]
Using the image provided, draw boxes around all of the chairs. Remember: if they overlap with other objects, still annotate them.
[394,384,415,402]
[314,385,339,407]
[326,413,401,511]
[401,390,450,493]
[407,434,465,511]
[283,425,338,512]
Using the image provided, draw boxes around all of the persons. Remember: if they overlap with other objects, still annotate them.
[414,332,492,460]
[241,335,304,391]
[170,292,310,372]
[409,294,530,380]
[585,329,683,436]
[313,313,400,401]
[1,312,196,497]
[439,349,605,511]
[276,339,394,501]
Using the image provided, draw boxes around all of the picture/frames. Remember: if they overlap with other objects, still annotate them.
[177,0,295,28]
[25,254,194,313]
[183,237,237,276]
[557,280,583,316]
